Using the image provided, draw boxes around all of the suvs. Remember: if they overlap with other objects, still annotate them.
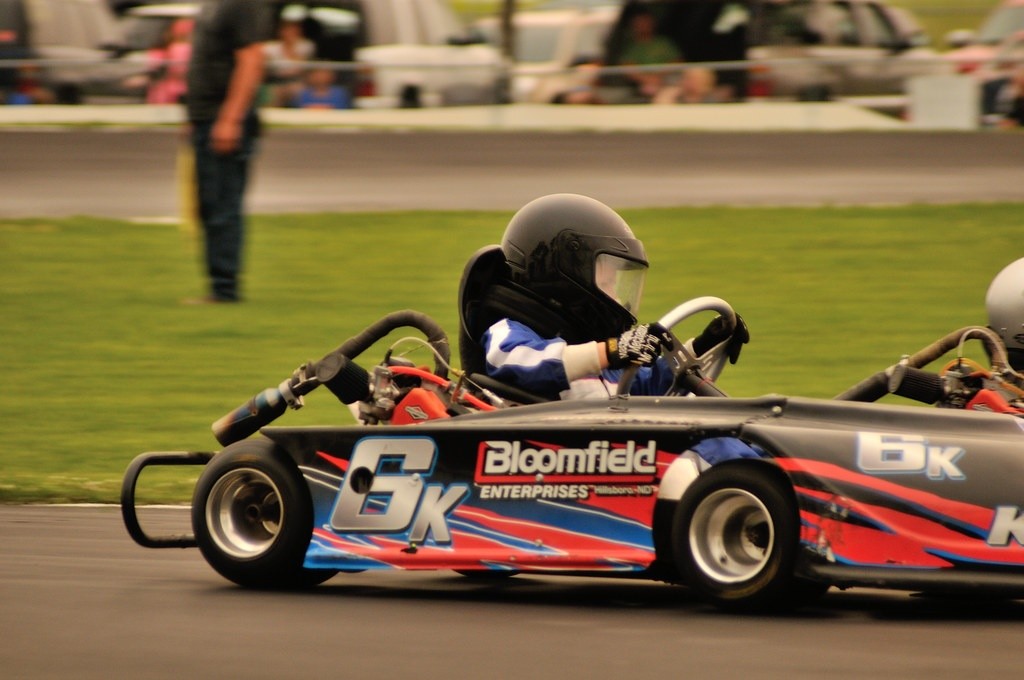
[263,0,515,108]
[559,1,935,119]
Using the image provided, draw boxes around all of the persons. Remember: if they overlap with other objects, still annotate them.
[0,22,79,104]
[185,1,266,302]
[261,21,353,110]
[968,255,1024,417]
[459,195,752,405]
[554,11,736,106]
[144,18,197,104]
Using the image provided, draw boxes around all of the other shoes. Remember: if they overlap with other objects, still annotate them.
[188,291,250,302]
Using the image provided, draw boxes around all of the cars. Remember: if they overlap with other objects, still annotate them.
[34,0,207,103]
[479,10,618,105]
[943,0,1024,124]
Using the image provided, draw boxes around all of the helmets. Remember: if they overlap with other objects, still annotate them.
[500,192,648,345]
[984,256,1024,349]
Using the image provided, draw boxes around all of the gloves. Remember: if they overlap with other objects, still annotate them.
[693,311,750,365]
[605,323,674,368]
